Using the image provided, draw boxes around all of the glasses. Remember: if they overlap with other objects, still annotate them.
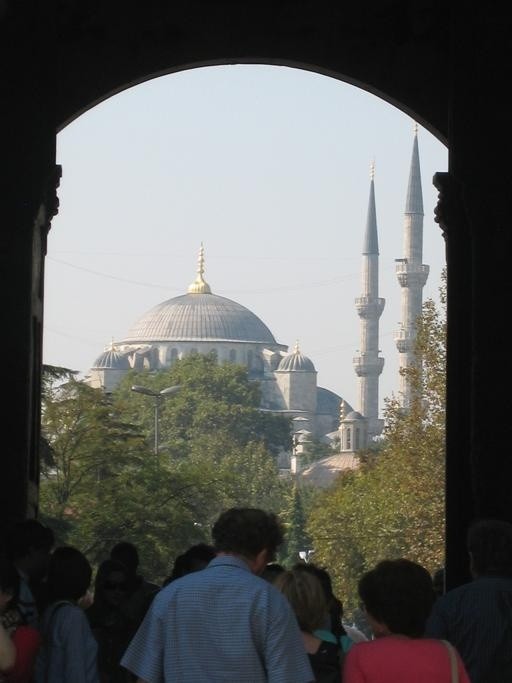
[103,579,128,591]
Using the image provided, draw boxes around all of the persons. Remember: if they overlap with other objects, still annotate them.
[1,520,511,683]
[119,507,318,683]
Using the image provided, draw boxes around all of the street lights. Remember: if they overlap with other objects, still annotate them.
[131,384,181,473]
